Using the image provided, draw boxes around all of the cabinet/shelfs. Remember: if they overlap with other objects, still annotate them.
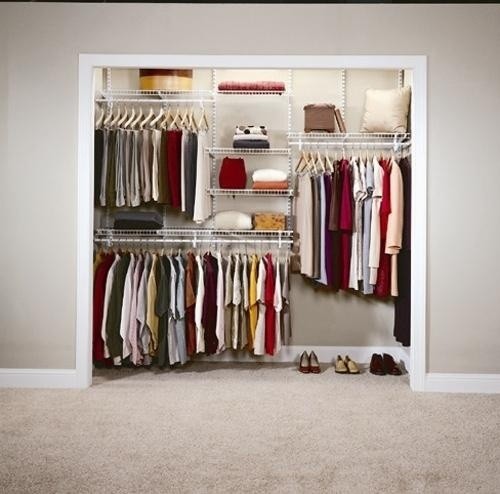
[205,87,298,229]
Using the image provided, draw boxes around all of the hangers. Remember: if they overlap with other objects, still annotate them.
[96,236,292,260]
[96,95,210,135]
[295,139,398,178]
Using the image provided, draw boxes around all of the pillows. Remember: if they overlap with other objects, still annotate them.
[360,86,412,136]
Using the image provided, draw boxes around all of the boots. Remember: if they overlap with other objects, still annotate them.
[369,353,402,376]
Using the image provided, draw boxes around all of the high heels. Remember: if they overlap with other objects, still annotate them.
[299,350,321,374]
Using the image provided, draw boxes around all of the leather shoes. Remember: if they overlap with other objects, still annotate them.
[335,355,360,374]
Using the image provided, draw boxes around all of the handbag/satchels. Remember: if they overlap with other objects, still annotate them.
[219,156,247,189]
